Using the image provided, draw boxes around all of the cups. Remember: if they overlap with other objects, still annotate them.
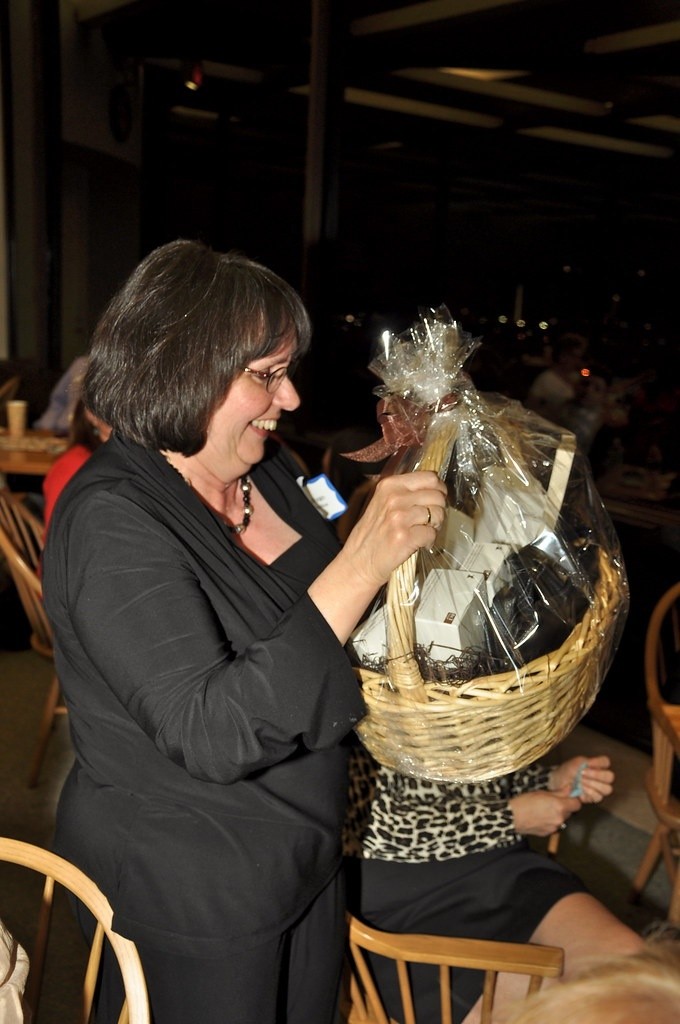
[6,400,26,435]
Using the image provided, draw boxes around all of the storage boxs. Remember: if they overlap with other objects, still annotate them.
[349,433,576,668]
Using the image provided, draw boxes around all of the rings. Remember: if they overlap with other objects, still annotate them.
[560,822,567,830]
[426,506,432,525]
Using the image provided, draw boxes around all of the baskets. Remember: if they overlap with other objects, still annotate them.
[349,414,631,783]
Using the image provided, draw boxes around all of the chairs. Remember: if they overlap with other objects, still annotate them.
[628,583,679,937]
[0,837,150,1024]
[0,483,67,787]
[336,830,564,1024]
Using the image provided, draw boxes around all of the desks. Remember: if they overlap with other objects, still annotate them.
[0,426,70,493]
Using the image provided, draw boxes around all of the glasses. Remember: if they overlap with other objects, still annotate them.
[240,365,288,393]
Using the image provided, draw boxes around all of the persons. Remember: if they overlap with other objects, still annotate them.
[41,239,450,1023]
[0,334,680,1023]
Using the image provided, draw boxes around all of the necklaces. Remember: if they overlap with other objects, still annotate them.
[167,460,253,535]
